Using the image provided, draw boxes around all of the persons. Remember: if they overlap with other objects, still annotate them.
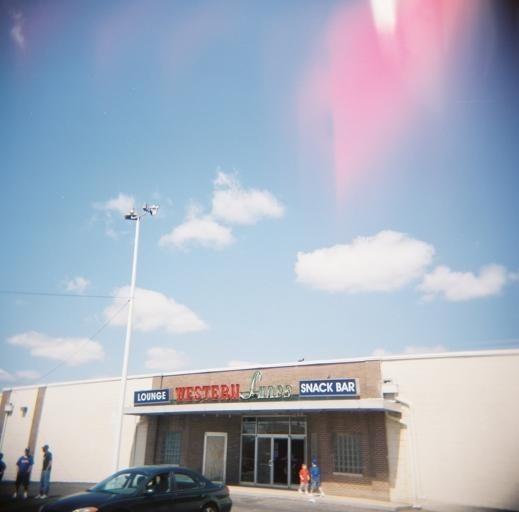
[0,453,6,483]
[298,464,310,494]
[11,448,34,500]
[309,459,325,497]
[34,445,53,500]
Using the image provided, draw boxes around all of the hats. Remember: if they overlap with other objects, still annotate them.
[312,460,318,465]
[25,448,30,452]
[42,445,49,449]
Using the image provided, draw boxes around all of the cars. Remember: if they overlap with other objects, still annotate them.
[39,465,231,512]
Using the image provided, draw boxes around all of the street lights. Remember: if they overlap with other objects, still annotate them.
[111,202,160,491]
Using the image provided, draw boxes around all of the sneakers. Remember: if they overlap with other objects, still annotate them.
[12,492,47,500]
